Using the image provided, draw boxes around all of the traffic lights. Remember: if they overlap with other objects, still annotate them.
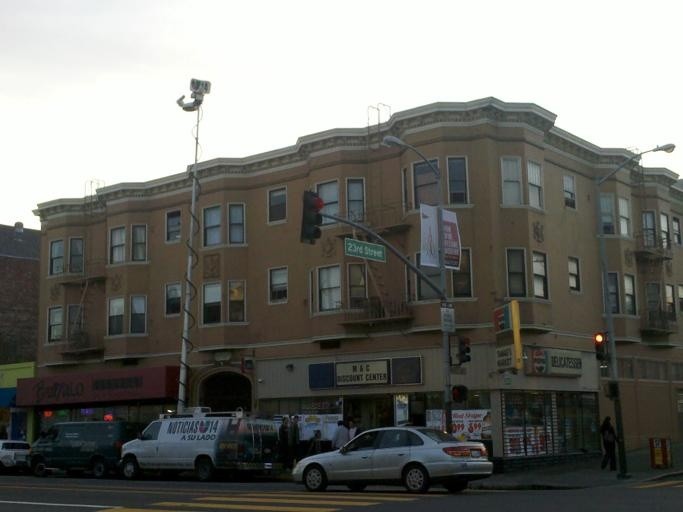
[452,384,468,404]
[605,379,618,402]
[456,331,473,368]
[593,332,614,369]
[300,189,324,246]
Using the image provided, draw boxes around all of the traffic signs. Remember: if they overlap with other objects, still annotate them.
[343,237,387,264]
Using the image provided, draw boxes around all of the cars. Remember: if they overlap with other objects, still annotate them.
[288,424,497,494]
[0,438,32,475]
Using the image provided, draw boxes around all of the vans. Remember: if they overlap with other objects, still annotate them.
[28,419,148,480]
[118,404,278,482]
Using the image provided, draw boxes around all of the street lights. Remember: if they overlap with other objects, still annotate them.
[594,140,675,481]
[379,132,457,435]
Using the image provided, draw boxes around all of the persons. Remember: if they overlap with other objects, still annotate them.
[277,415,300,473]
[600,415,618,472]
[348,420,366,449]
[308,430,322,454]
[330,420,349,452]
